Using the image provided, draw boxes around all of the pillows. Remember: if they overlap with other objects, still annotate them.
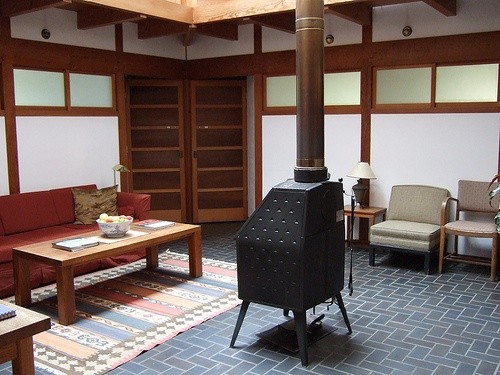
[71,185,118,225]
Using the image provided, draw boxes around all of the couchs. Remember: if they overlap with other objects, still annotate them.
[0,184,151,298]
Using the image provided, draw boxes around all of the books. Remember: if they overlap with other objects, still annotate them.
[133,219,176,231]
[50,237,99,253]
[0,303,17,320]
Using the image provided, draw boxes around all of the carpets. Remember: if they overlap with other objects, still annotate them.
[3,248,243,375]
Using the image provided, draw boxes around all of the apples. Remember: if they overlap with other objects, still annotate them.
[99,212,133,223]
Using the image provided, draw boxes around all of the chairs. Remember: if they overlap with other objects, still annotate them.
[369,184,450,274]
[439,180,500,282]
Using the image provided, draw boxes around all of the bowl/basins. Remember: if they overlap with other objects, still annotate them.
[96,216,134,237]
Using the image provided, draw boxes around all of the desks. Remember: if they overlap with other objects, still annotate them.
[0,299,51,375]
[11,218,202,327]
[344,205,387,248]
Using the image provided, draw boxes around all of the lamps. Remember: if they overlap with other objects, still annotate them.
[346,162,376,209]
[112,164,126,185]
